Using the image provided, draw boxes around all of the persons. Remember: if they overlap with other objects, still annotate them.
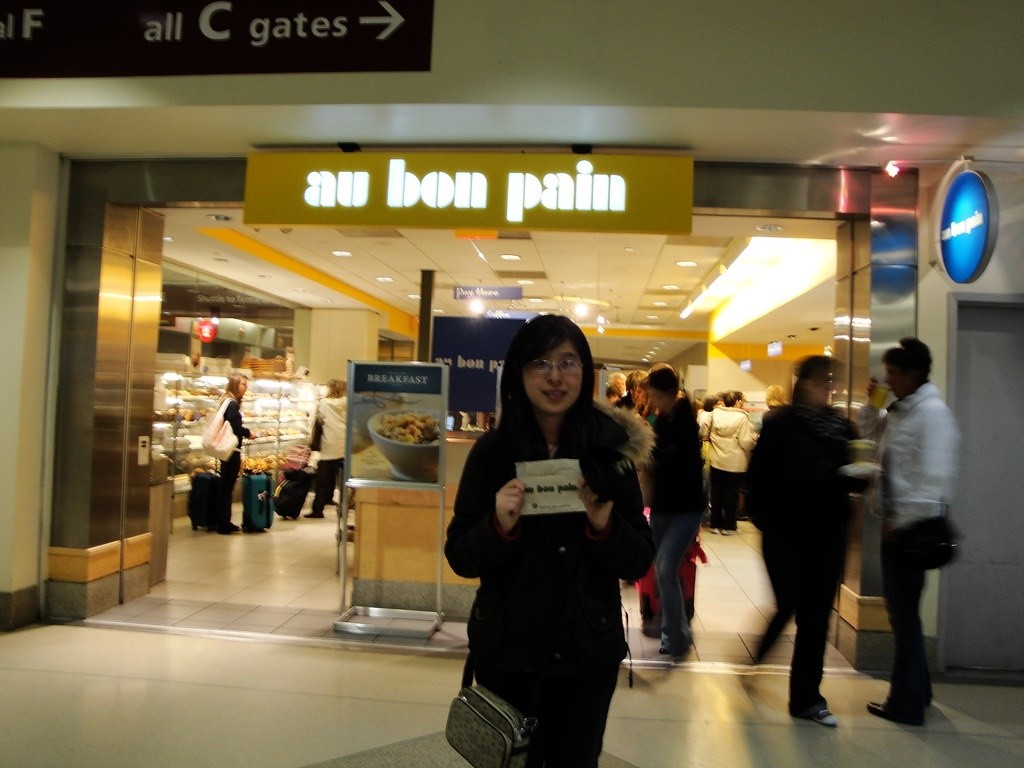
[203,367,258,534]
[856,338,960,729]
[604,366,788,660]
[308,378,351,519]
[444,316,656,768]
[739,352,873,726]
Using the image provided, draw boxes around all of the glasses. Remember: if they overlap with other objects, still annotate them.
[527,360,584,373]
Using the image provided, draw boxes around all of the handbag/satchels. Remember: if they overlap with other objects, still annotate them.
[890,516,957,571]
[201,398,242,462]
[446,684,539,768]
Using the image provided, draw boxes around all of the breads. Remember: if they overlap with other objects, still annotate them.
[153,389,307,473]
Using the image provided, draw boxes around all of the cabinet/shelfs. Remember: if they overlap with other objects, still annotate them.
[153,372,316,535]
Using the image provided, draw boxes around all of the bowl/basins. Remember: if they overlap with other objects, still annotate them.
[368,409,440,481]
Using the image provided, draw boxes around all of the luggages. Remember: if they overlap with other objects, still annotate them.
[274,451,316,520]
[635,558,696,636]
[187,457,220,532]
[242,438,274,532]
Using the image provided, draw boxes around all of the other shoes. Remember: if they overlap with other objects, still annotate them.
[304,512,324,518]
[866,702,925,726]
[709,528,743,535]
[813,710,838,724]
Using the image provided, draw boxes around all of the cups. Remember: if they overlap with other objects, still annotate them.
[869,381,889,408]
